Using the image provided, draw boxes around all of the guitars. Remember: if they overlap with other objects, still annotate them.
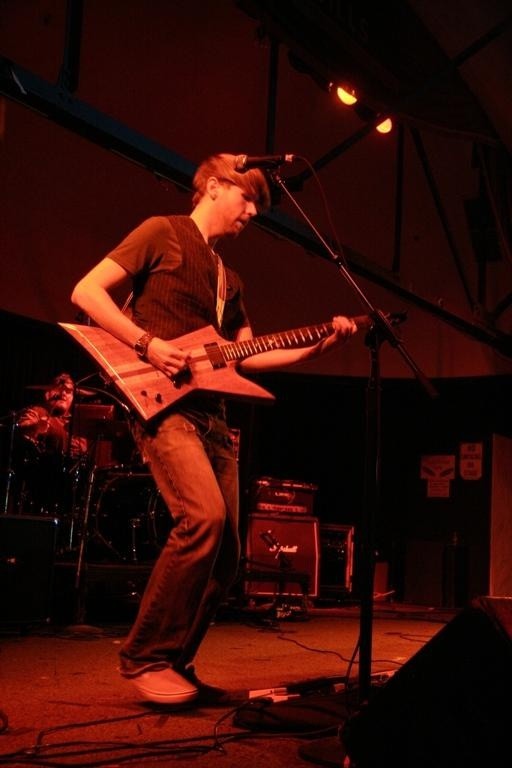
[56,307,405,424]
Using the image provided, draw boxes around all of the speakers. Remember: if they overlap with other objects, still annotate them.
[366,595,512,768]
[243,513,321,598]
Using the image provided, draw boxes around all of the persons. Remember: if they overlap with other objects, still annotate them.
[70,152,359,705]
[18,373,88,469]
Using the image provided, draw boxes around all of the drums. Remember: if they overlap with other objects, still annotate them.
[94,474,163,564]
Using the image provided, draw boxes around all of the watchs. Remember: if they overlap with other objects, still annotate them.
[134,331,156,361]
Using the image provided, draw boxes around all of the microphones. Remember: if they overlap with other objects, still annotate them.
[232,153,296,173]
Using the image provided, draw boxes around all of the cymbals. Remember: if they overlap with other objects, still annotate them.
[33,382,94,395]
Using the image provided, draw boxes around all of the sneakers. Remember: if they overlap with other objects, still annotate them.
[119,667,198,704]
[187,678,231,704]
